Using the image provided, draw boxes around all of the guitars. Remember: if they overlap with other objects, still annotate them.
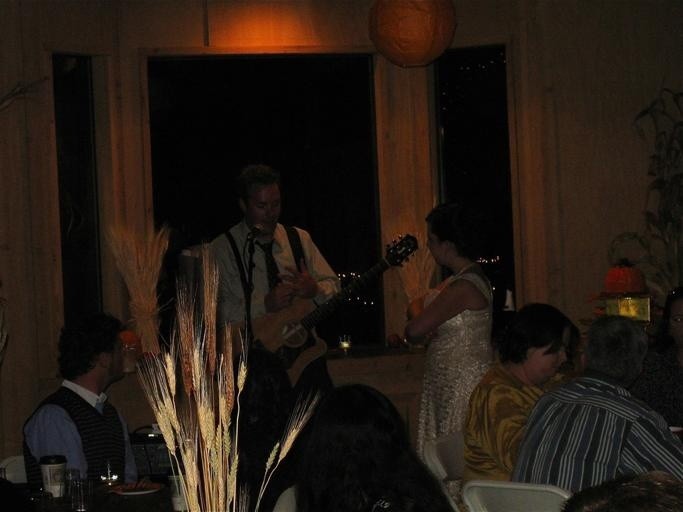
[216,233,419,398]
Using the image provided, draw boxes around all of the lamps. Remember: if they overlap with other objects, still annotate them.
[592,257,658,326]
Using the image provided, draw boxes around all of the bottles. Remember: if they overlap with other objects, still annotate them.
[502,288,515,323]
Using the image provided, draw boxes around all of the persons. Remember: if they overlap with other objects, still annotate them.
[200,162,344,512]
[23,315,138,493]
[464,286,683,512]
[301,384,454,510]
[404,199,497,487]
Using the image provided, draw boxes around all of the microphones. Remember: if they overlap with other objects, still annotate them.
[246,223,265,254]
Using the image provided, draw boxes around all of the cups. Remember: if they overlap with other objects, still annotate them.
[37,453,66,498]
[118,330,137,374]
[167,474,189,511]
[53,469,80,501]
[338,336,351,356]
[69,478,91,511]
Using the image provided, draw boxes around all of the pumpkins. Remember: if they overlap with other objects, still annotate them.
[604,257,646,293]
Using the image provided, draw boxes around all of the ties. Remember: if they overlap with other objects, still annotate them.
[253,242,280,286]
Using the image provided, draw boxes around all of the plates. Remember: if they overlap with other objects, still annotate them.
[110,483,162,495]
